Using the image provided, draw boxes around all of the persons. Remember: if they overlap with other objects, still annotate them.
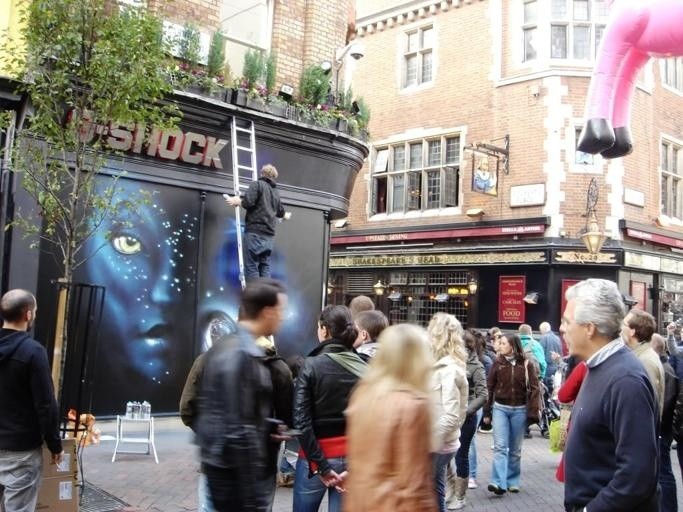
[0,289,63,511]
[84,177,192,388]
[179,279,683,510]
[473,157,497,195]
[224,164,291,285]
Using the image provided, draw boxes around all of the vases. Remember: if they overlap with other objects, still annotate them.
[234,91,288,118]
[297,109,338,130]
[339,120,354,133]
[173,76,232,104]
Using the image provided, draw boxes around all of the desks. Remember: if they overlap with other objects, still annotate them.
[111,416,159,463]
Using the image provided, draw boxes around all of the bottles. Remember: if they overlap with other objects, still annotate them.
[124,400,150,417]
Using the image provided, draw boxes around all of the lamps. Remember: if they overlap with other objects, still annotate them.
[658,283,665,301]
[327,280,335,296]
[436,294,449,303]
[467,278,479,295]
[466,209,485,218]
[523,293,543,306]
[387,292,403,302]
[337,221,350,231]
[372,280,389,297]
[655,215,674,230]
[578,178,610,255]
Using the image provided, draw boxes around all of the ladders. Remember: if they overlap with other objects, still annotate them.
[230,116,274,347]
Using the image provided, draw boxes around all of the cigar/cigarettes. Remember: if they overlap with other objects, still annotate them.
[54,462,60,470]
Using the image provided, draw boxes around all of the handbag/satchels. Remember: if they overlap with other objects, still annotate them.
[527,382,547,416]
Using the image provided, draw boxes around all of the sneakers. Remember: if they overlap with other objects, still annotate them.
[487,484,505,495]
[509,486,519,492]
[444,493,468,510]
[468,480,477,488]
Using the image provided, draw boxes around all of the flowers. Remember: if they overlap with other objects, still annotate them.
[293,101,336,129]
[338,112,365,133]
[238,75,285,102]
[163,64,224,89]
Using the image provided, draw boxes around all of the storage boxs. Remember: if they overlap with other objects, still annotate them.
[35,440,80,512]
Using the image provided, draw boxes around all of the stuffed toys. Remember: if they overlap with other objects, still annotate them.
[575,1,682,159]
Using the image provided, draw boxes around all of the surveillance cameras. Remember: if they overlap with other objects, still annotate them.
[350,44,364,60]
